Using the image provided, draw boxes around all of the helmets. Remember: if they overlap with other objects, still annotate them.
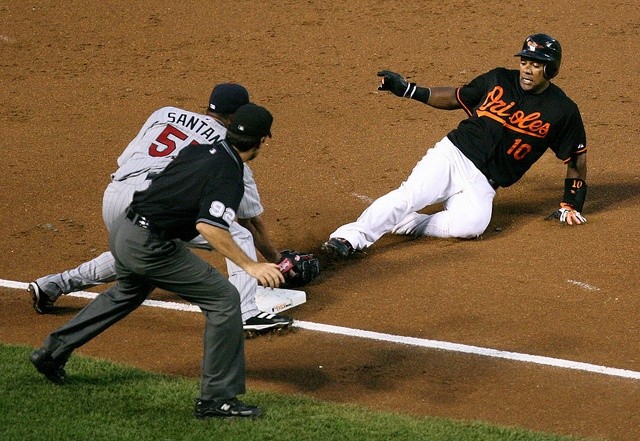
[514,33,562,81]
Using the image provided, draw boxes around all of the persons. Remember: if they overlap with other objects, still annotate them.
[30,104,286,418]
[27,83,294,331]
[323,32,588,260]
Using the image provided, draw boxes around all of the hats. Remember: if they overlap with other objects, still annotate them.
[231,103,275,143]
[209,81,255,114]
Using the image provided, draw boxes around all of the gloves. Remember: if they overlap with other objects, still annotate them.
[280,249,317,280]
[375,70,431,107]
[544,202,588,226]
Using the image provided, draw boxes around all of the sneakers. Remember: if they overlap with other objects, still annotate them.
[241,312,293,331]
[25,279,52,314]
[193,396,261,416]
[319,236,357,260]
[30,347,67,380]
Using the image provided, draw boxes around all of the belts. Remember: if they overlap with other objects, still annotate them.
[125,206,159,232]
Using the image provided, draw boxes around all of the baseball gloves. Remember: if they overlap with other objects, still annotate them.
[278,250,321,289]
